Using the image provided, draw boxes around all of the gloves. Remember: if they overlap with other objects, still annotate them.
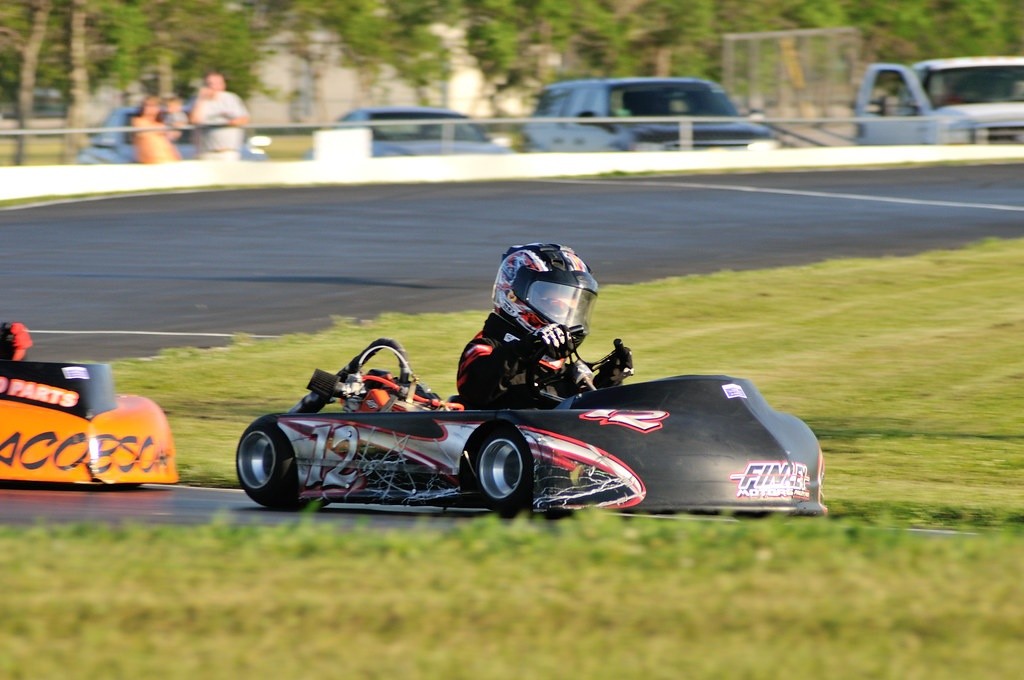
[600,347,634,386]
[518,322,574,362]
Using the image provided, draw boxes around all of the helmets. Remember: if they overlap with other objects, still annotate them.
[492,243,597,349]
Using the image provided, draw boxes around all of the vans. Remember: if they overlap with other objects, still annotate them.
[851,55,1024,146]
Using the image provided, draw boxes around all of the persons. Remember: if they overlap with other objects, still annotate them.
[456,244,635,409]
[129,96,184,161]
[189,71,249,158]
[877,73,912,113]
[164,96,187,137]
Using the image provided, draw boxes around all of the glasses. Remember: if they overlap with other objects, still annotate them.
[538,296,583,324]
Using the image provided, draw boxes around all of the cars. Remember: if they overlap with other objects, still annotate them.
[303,105,515,162]
[521,75,769,151]
[73,103,270,164]
